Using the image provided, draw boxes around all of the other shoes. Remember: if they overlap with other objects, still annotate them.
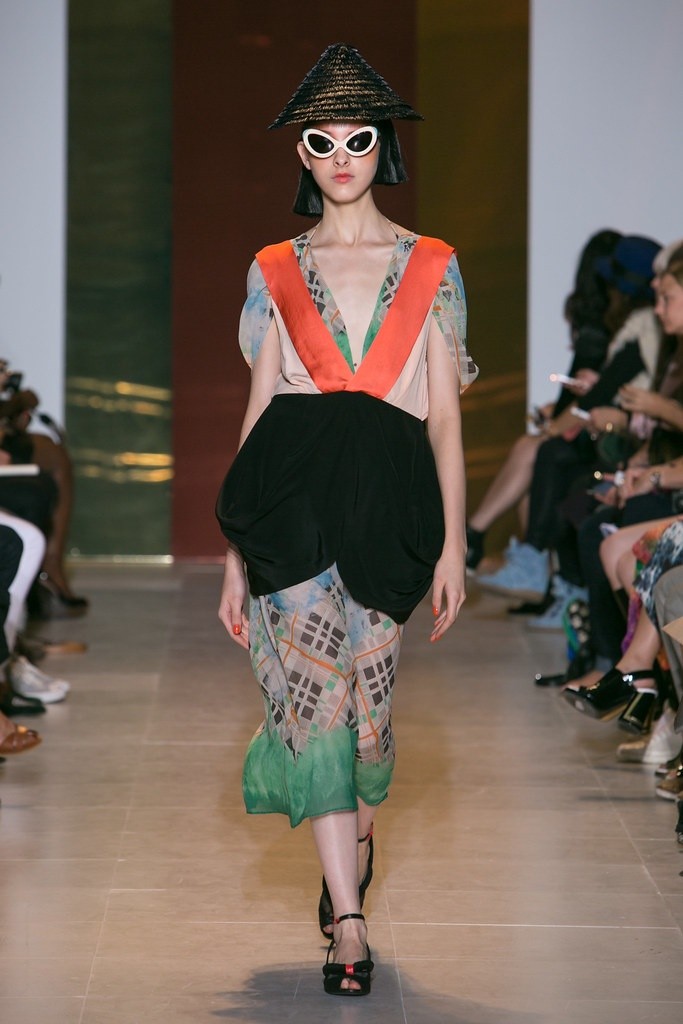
[465,525,484,576]
[508,596,553,614]
[568,672,604,690]
[656,756,681,773]
[657,776,683,800]
[9,655,70,702]
[536,644,593,688]
[0,690,44,713]
[618,734,650,760]
[0,722,41,753]
[32,570,89,608]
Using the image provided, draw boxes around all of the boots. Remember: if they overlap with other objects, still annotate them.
[477,535,548,601]
[528,576,588,628]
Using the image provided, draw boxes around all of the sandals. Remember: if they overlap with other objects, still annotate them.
[319,821,373,938]
[323,915,374,996]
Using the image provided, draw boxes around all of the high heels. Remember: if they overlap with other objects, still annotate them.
[562,668,658,735]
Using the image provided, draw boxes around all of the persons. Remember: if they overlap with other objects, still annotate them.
[0,359,89,757]
[215,40,480,996]
[469,229,683,802]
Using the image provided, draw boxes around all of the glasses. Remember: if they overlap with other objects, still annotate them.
[297,125,382,158]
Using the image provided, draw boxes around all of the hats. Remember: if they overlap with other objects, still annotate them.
[267,43,425,129]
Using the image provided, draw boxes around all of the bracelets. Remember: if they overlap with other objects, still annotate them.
[651,470,662,491]
